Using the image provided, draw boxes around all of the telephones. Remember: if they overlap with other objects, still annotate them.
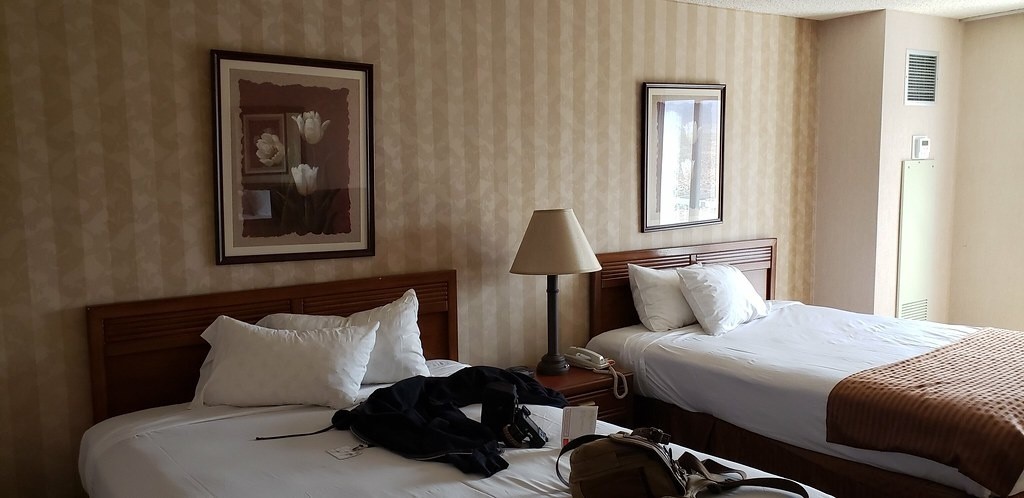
[563,346,616,370]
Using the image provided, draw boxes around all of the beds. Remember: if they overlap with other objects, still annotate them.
[78,266,834,498]
[589,237,1024,498]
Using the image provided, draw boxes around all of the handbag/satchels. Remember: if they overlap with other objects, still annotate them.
[556,434,810,498]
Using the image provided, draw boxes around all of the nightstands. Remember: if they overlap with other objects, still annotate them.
[525,361,637,429]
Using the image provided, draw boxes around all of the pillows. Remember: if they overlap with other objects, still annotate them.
[675,263,770,335]
[256,288,429,384]
[187,315,380,409]
[627,262,702,332]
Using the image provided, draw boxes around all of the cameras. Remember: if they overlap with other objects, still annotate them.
[501,405,550,450]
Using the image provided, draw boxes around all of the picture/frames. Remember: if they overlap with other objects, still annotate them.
[642,82,726,234]
[210,48,375,265]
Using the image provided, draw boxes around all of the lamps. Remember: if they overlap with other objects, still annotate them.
[510,208,602,376]
